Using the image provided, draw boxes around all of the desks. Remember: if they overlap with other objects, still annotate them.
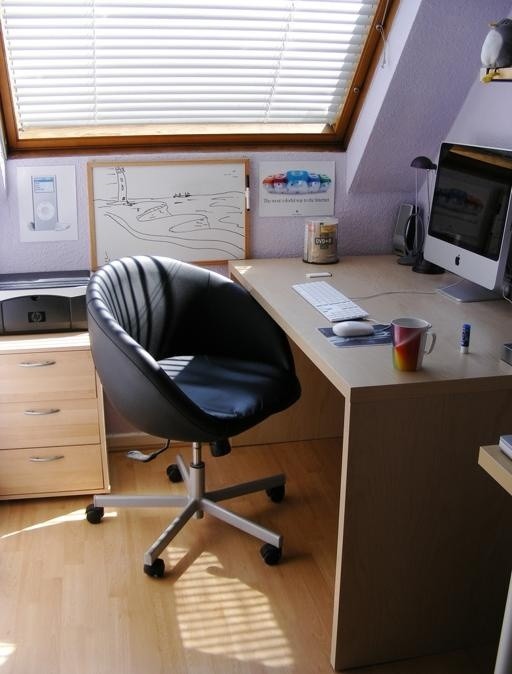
[477,443,512,670]
[226,252,512,673]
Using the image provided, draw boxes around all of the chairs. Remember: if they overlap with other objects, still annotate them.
[85,254,302,579]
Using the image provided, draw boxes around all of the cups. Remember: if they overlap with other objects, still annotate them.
[388,316,436,374]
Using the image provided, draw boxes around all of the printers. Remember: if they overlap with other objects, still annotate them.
[0,274,95,335]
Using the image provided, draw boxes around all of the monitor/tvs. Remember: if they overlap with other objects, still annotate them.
[421,142,512,302]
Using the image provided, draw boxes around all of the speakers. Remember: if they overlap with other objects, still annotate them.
[390,203,418,256]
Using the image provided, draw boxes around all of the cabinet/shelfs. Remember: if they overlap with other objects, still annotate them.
[0,331,112,500]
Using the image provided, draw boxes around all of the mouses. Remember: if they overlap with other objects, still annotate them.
[332,320,375,337]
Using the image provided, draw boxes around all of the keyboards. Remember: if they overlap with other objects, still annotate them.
[292,280,369,322]
